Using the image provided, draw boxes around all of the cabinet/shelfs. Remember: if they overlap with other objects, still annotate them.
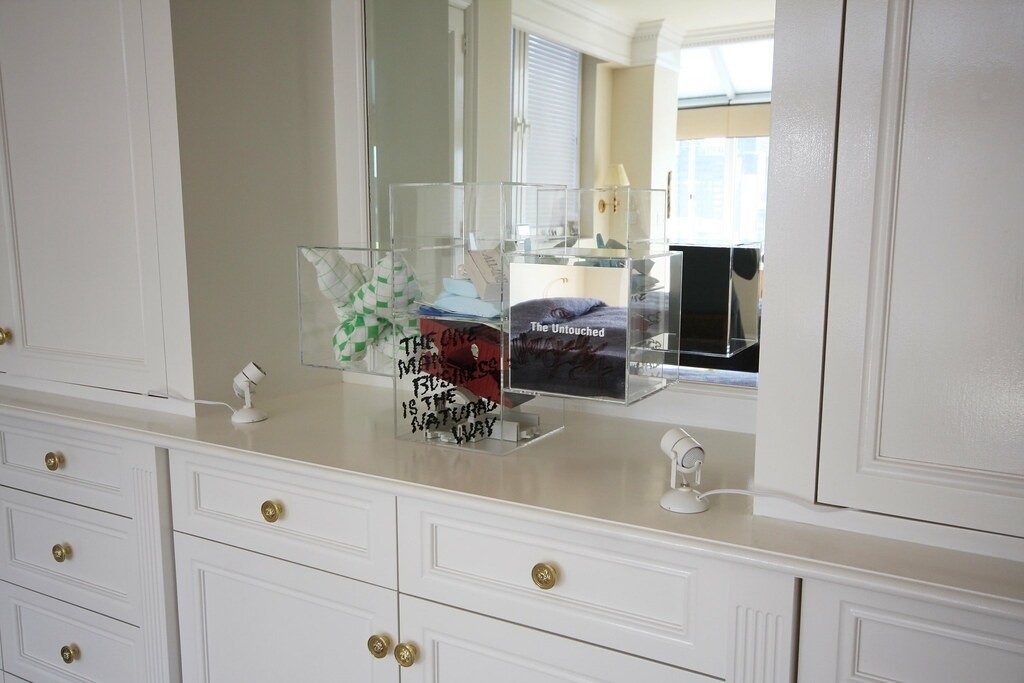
[0,0,1024,683]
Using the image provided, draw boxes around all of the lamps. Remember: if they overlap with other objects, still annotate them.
[655,423,709,515]
[227,356,273,426]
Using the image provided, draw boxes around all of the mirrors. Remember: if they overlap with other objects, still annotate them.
[330,0,779,436]
